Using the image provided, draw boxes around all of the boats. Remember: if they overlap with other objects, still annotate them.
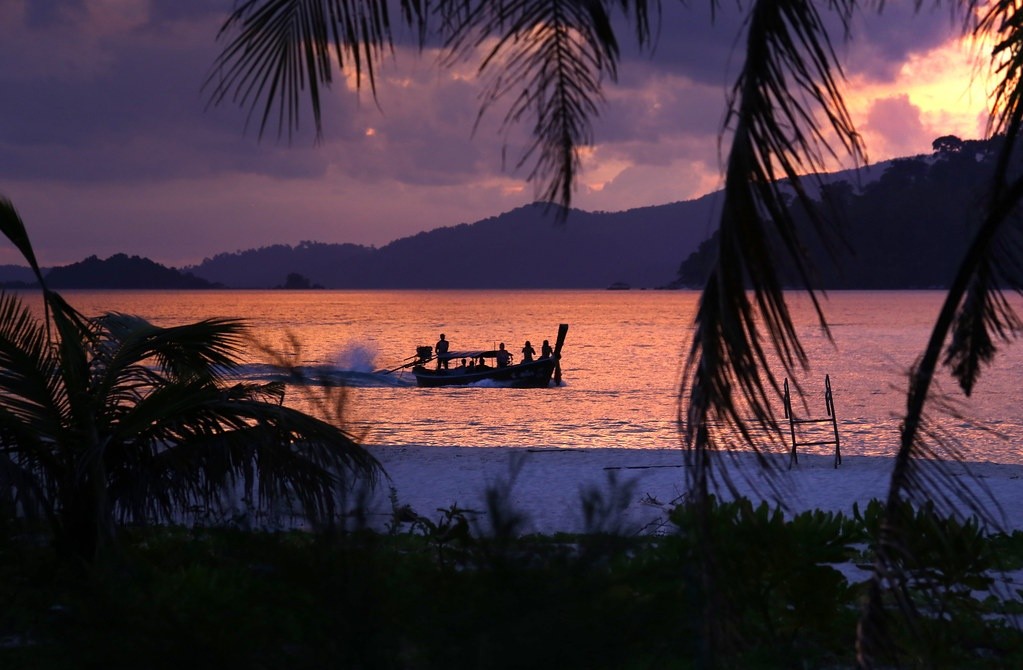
[412,324,568,388]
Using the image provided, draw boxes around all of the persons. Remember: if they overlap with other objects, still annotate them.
[435,334,449,369]
[542,340,554,359]
[521,341,535,362]
[456,358,489,370]
[496,343,509,368]
